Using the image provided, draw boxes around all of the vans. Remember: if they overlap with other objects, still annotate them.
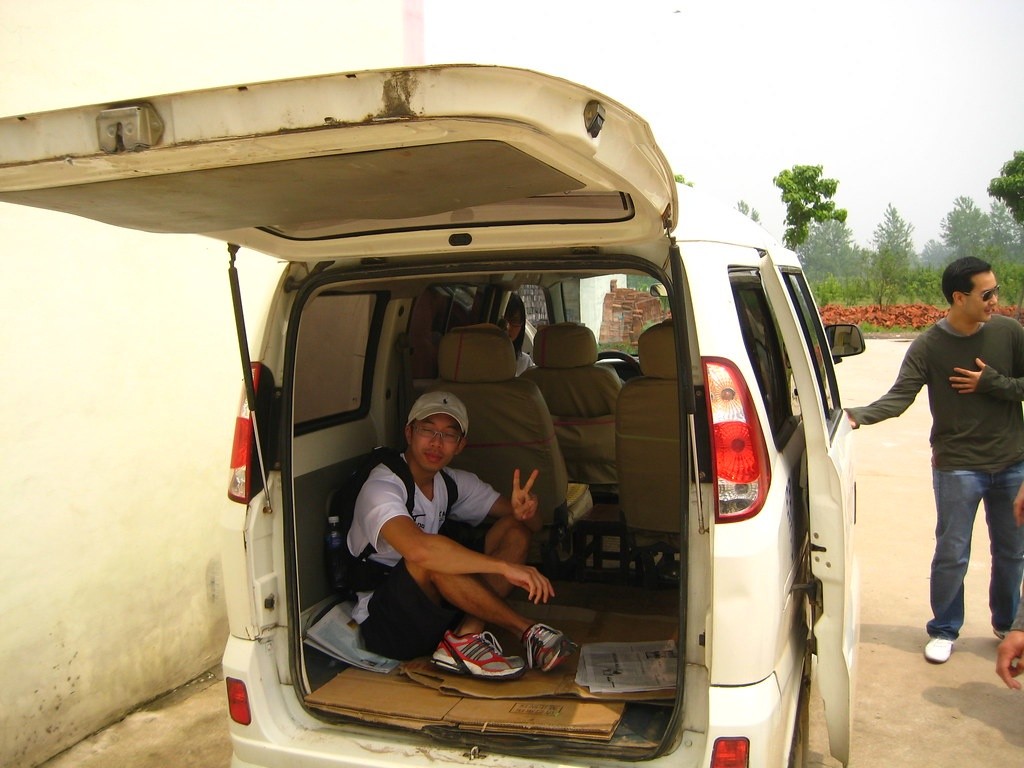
[0,59,868,768]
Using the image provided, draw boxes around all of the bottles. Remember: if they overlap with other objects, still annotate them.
[326,517,343,587]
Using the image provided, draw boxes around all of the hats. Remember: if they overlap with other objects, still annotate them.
[408,391,469,433]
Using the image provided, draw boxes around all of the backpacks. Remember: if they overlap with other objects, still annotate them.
[319,445,459,603]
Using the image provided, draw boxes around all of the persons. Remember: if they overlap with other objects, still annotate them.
[501,292,536,378]
[1007,478,1024,530]
[347,389,572,682]
[841,256,1024,664]
[994,570,1024,692]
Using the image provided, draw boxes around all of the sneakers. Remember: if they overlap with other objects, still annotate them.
[925,638,954,663]
[993,626,1010,640]
[521,622,570,671]
[430,629,527,681]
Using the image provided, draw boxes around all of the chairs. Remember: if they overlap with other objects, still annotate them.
[518,322,621,485]
[414,324,593,564]
[613,322,681,533]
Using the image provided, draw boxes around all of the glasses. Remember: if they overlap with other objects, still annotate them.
[505,317,523,327]
[964,284,1000,302]
[410,424,462,442]
